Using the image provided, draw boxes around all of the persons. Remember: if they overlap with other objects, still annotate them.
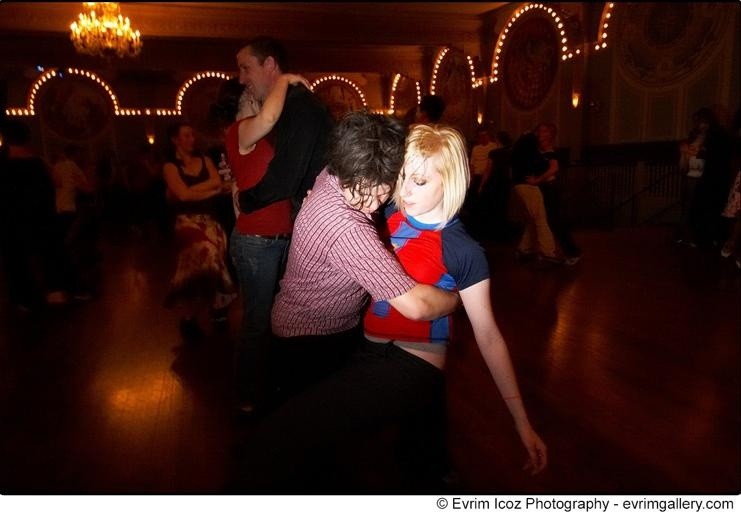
[228,36,338,236]
[360,124,550,494]
[269,108,460,493]
[162,123,238,318]
[676,116,740,298]
[469,122,586,267]
[207,73,316,419]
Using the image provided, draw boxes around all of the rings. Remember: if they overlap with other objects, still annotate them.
[538,454,543,457]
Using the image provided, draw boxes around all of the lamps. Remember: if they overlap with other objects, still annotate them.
[70,2,144,60]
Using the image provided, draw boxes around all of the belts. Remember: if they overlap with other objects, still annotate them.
[245,233,285,240]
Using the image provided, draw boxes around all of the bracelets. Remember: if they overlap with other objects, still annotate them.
[501,394,525,402]
